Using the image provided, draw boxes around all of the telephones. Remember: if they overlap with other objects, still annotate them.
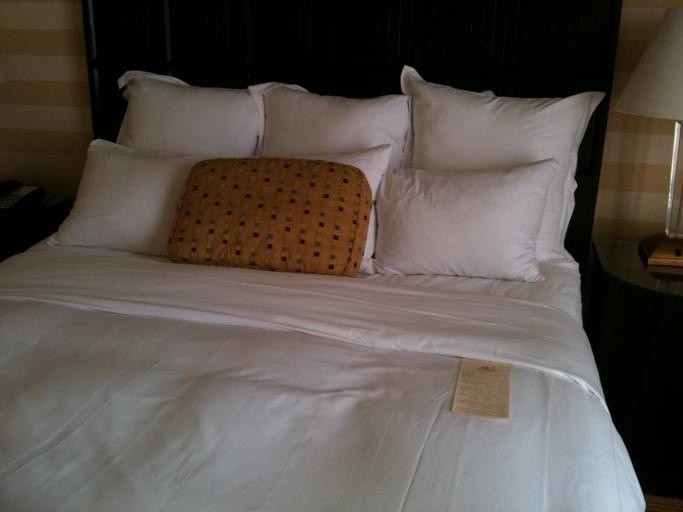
[2,179,45,226]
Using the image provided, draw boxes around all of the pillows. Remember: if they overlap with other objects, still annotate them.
[249,79,408,194]
[54,138,192,257]
[372,161,557,284]
[116,71,261,163]
[167,157,373,278]
[166,142,394,275]
[399,63,604,270]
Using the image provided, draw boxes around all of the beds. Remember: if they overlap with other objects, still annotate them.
[0,0,647,512]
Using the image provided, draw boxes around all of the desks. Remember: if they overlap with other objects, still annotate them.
[585,236,683,364]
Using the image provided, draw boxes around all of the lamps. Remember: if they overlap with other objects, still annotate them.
[614,1,682,274]
